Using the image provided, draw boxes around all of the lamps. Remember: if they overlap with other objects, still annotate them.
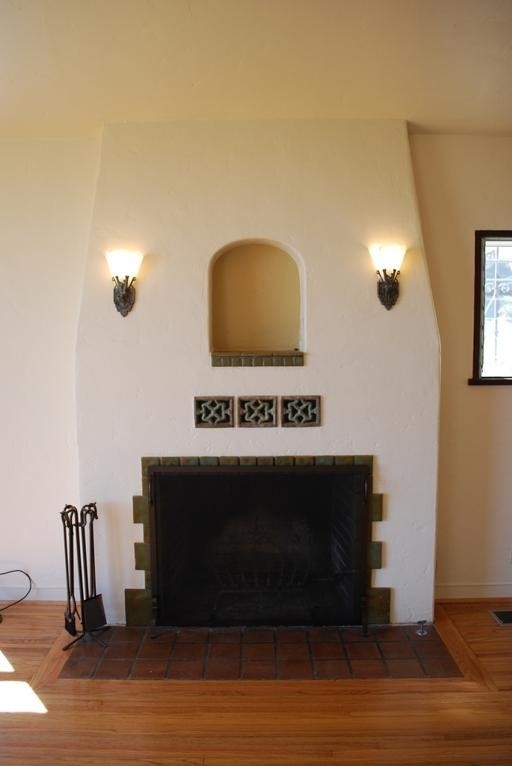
[106,249,144,319]
[368,242,406,311]
